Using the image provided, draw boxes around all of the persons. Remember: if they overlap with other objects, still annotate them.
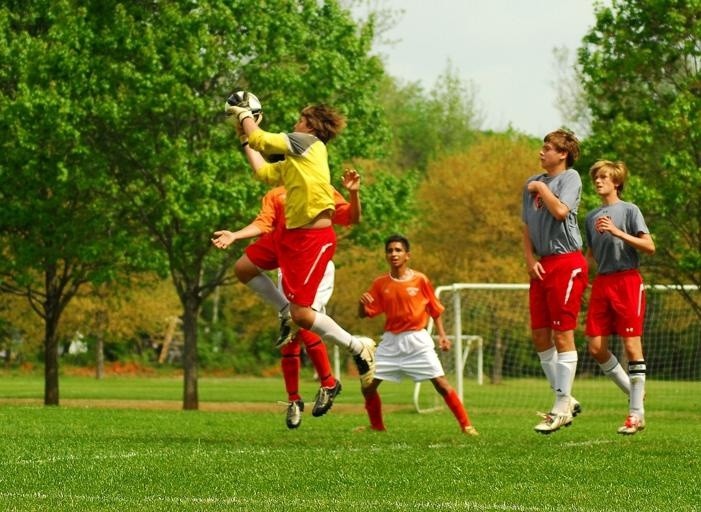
[588,160,658,435]
[519,126,588,435]
[210,170,360,428]
[361,234,480,438]
[224,88,377,392]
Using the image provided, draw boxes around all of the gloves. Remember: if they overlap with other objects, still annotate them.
[235,122,250,146]
[227,90,254,123]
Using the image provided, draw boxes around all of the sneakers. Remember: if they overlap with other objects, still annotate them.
[572,400,580,416]
[312,380,341,417]
[271,317,299,348]
[287,401,304,429]
[353,337,376,389]
[351,426,386,434]
[534,413,572,433]
[462,425,478,435]
[617,417,646,435]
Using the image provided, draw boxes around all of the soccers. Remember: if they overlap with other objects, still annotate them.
[225,89,261,125]
